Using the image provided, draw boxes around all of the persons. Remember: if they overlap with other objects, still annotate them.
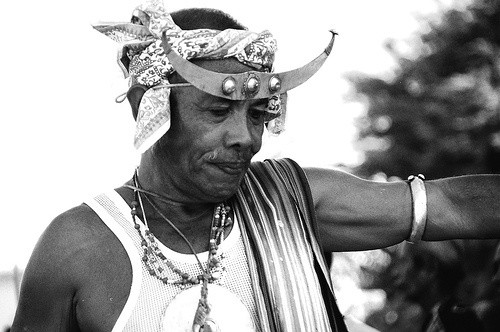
[10,10,500,331]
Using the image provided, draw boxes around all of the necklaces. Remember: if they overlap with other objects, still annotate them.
[131,166,230,287]
